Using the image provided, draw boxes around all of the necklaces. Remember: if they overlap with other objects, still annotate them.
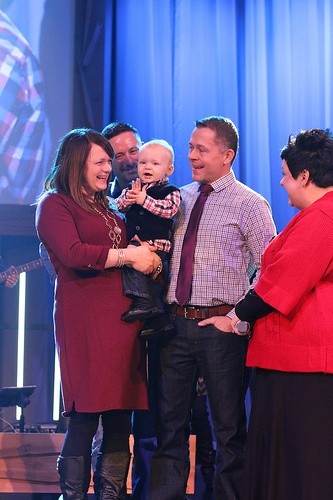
[92,202,122,250]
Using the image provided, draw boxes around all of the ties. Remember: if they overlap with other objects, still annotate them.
[175,183,213,308]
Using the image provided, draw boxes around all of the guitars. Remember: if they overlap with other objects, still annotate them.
[0,258,44,282]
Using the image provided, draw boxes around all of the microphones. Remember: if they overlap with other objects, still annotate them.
[235,320,249,333]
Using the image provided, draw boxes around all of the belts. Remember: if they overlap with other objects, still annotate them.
[174,305,235,320]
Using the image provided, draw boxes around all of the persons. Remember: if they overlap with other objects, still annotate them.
[1,263,21,289]
[158,115,280,498]
[114,140,182,324]
[90,121,161,499]
[32,128,163,500]
[230,127,333,499]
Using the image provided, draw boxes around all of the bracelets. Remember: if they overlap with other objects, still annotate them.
[157,259,162,272]
[117,248,125,268]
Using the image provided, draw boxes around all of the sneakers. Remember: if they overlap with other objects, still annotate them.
[122,300,160,323]
[140,313,174,337]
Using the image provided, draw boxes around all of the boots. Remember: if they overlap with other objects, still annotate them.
[93,452,132,500]
[58,455,91,500]
